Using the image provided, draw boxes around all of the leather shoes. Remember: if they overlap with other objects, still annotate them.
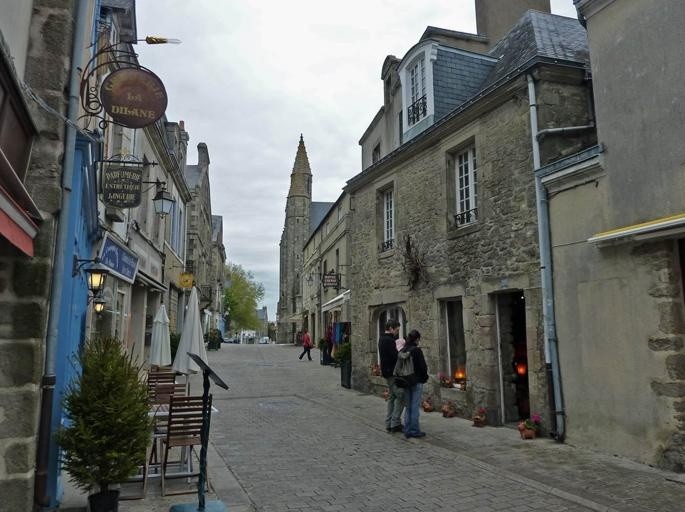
[385,424,427,439]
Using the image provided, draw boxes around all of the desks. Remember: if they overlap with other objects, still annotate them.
[127,401,219,480]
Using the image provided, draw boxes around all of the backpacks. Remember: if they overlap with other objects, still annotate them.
[393,348,417,389]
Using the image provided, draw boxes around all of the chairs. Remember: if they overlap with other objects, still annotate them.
[145,362,212,497]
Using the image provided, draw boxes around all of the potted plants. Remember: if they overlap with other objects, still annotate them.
[335,340,351,389]
[54,328,152,511]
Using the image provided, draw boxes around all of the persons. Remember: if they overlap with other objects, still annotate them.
[325,326,349,353]
[378,317,405,433]
[298,329,314,361]
[233,331,273,345]
[399,329,428,439]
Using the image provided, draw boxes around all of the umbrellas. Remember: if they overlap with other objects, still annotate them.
[172,283,211,483]
[146,304,173,404]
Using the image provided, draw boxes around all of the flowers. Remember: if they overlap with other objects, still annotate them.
[424,373,544,430]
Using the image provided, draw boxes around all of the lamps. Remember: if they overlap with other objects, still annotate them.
[72,249,107,314]
[142,181,175,218]
